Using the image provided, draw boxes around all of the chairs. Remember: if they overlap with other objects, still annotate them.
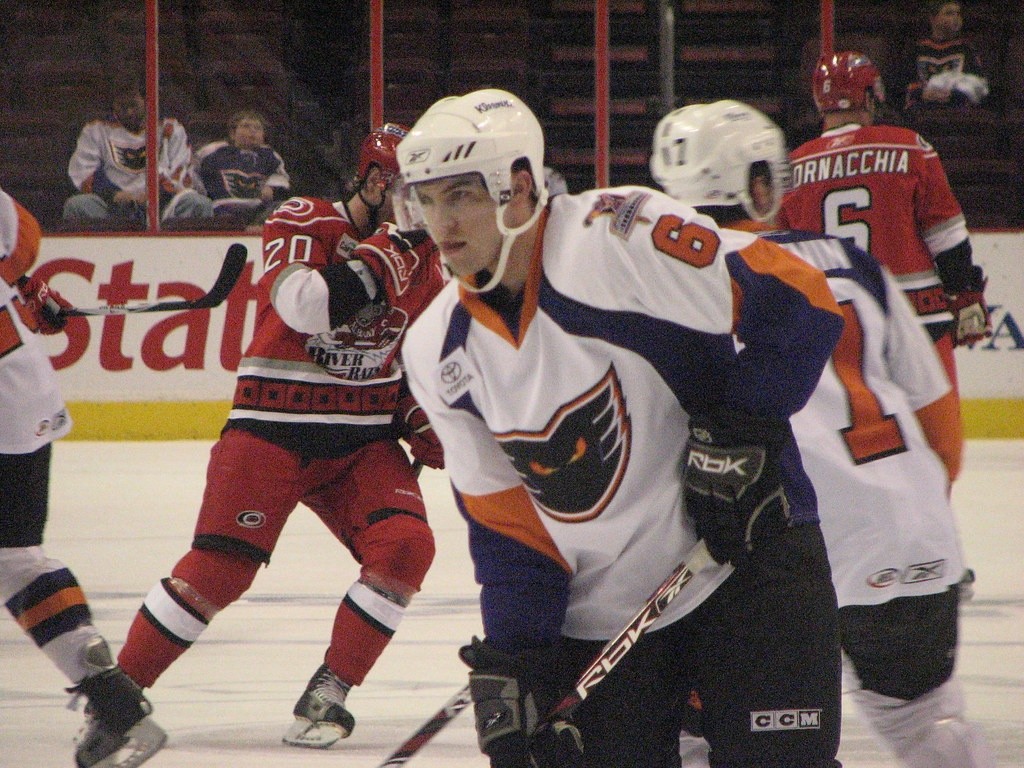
[0,0,1024,240]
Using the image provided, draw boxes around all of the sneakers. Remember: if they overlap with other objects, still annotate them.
[282,664,356,748]
[64,664,168,768]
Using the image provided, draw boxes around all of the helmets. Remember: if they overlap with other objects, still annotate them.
[395,88,546,204]
[354,122,411,191]
[647,98,787,222]
[811,52,884,116]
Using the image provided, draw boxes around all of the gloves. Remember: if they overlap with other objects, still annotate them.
[458,636,584,768]
[394,384,445,469]
[352,220,428,301]
[674,414,793,571]
[944,264,992,349]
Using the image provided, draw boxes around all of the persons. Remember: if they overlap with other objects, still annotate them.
[902,0,995,111]
[63,81,214,231]
[198,113,291,228]
[780,52,991,489]
[402,88,843,768]
[116,123,444,738]
[0,189,168,768]
[639,99,991,768]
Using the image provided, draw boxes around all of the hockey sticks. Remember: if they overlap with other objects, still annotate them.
[542,539,714,722]
[381,684,471,768]
[59,243,249,317]
